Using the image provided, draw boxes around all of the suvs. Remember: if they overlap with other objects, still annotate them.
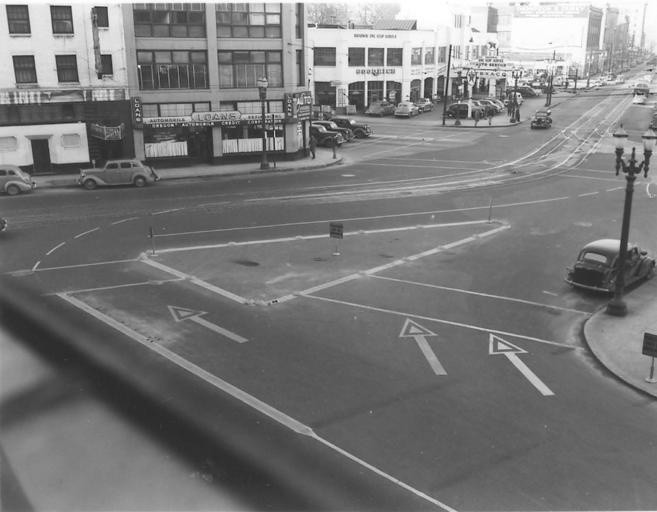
[530,110,554,129]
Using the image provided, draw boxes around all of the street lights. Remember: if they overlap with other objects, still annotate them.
[256,75,268,170]
[600,53,607,78]
[572,58,580,91]
[509,70,522,123]
[585,56,593,87]
[603,122,656,314]
[547,42,556,63]
[543,59,558,105]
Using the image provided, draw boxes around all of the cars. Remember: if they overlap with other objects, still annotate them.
[308,105,370,149]
[365,98,435,119]
[75,158,161,190]
[594,70,630,85]
[623,53,656,74]
[0,163,38,197]
[441,98,505,121]
[506,85,538,105]
[566,238,654,297]
[524,71,569,90]
[0,215,6,234]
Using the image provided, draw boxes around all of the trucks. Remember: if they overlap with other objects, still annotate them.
[632,79,651,100]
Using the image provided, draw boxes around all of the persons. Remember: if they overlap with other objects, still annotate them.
[309,135,317,160]
[506,101,512,117]
[487,107,494,126]
[473,108,480,127]
